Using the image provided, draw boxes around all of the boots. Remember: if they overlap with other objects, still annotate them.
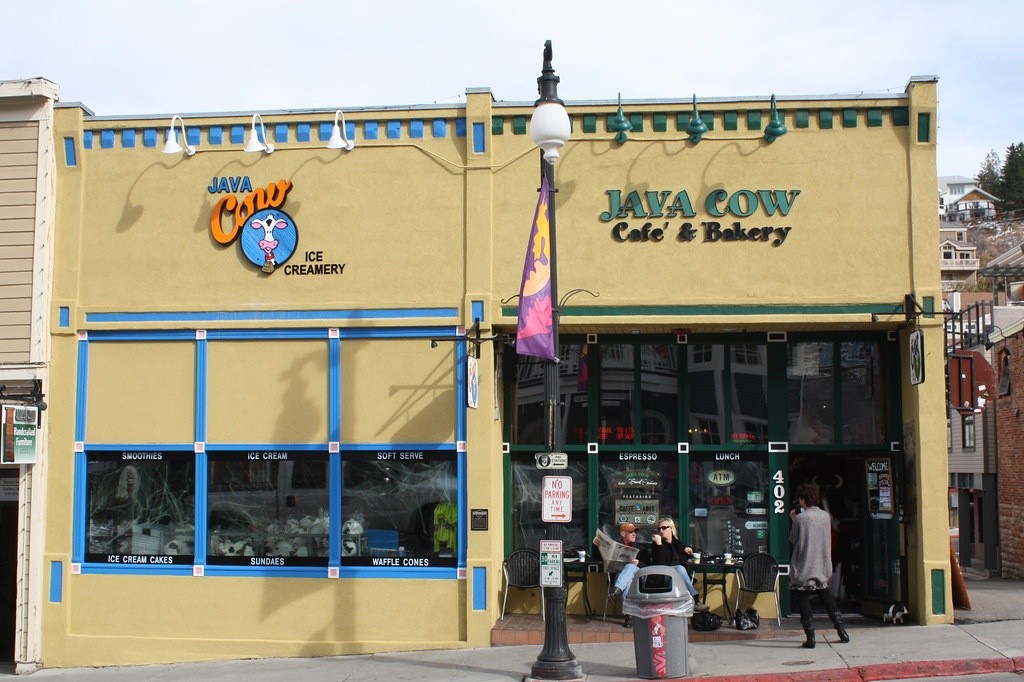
[829,611,850,643]
[609,587,622,603]
[802,618,816,648]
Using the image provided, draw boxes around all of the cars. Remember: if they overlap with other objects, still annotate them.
[181,452,460,547]
[511,462,591,519]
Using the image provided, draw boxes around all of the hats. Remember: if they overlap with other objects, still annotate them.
[620,522,639,531]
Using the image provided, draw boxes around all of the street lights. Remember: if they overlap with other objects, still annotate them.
[531,40,583,680]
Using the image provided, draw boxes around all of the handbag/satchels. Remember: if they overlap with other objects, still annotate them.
[691,610,723,630]
[736,607,759,629]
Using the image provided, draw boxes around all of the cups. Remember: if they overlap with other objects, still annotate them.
[693,553,701,564]
[577,550,585,562]
[724,553,732,564]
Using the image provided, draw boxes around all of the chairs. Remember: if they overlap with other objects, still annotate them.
[602,572,618,622]
[733,552,784,626]
[501,549,546,622]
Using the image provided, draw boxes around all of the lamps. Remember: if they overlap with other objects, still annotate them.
[162,115,195,156]
[686,93,708,144]
[764,93,787,143]
[326,109,354,150]
[985,326,1018,352]
[610,92,633,144]
[244,113,275,153]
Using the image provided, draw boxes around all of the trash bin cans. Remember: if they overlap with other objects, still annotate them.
[627,565,692,679]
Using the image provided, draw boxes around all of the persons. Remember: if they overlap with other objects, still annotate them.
[593,522,649,628]
[789,484,850,649]
[651,517,711,611]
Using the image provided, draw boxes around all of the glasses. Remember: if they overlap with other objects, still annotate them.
[628,530,637,533]
[658,526,669,531]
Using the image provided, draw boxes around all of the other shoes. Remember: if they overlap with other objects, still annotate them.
[695,601,710,610]
[625,613,632,628]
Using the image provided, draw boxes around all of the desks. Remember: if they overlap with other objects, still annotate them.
[563,561,596,620]
[686,560,737,628]
